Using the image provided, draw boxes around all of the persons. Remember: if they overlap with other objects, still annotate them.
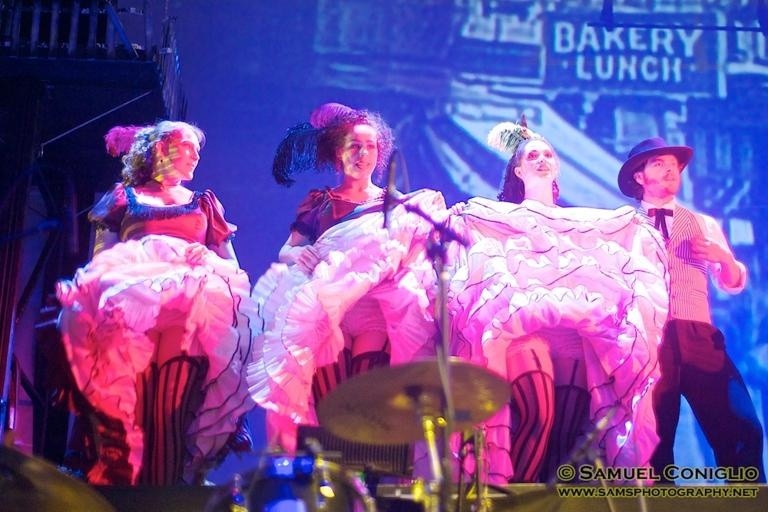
[616,135,768,485]
[54,120,265,488]
[448,114,672,485]
[243,102,449,451]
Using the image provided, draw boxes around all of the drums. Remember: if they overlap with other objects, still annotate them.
[204,455,379,511]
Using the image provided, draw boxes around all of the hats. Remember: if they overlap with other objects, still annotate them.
[618,138,693,200]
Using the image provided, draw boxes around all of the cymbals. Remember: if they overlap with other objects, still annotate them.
[0,444,112,511]
[317,360,512,446]
